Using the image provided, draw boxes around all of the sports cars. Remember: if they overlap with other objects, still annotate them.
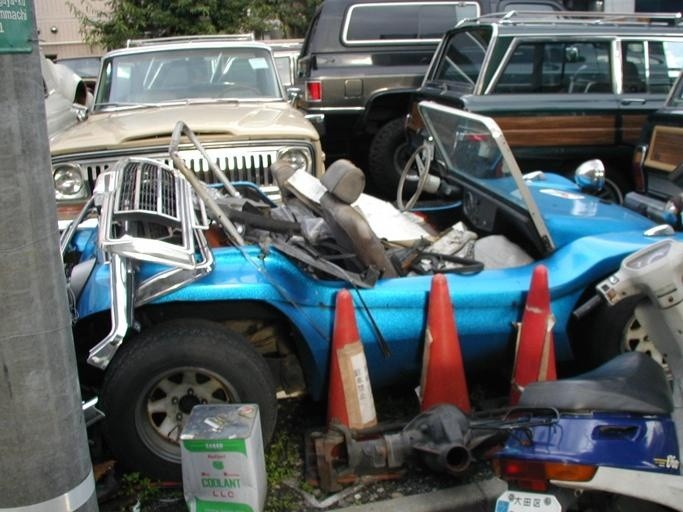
[57,99,677,483]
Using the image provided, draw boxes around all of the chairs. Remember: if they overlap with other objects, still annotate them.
[269,158,398,279]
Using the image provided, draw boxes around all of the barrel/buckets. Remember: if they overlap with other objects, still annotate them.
[181,402,268,512]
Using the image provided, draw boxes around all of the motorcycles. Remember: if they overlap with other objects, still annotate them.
[467,236,682,512]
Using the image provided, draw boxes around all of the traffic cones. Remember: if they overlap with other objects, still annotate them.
[413,271,470,413]
[507,263,558,418]
[313,287,398,482]
